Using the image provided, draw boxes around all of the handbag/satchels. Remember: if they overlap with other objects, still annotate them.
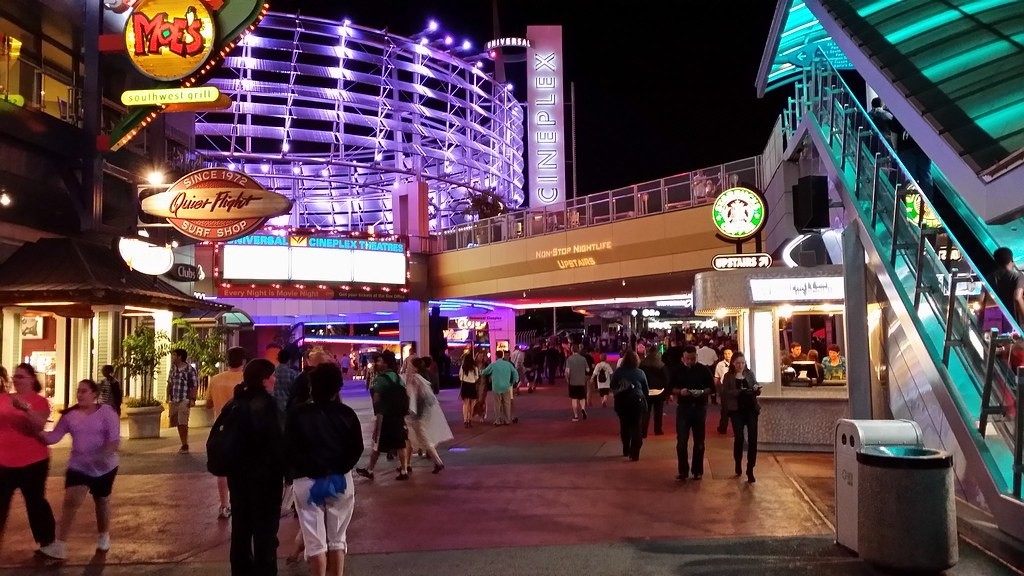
[458,381,463,400]
[206,414,230,478]
[612,364,634,396]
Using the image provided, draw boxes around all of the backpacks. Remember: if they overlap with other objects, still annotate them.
[599,363,608,382]
[377,373,411,418]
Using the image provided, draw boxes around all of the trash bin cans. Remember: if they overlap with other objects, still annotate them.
[834,418,923,556]
[856,446,960,576]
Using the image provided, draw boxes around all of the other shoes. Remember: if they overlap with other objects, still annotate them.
[431,463,445,474]
[96,534,110,550]
[180,445,189,453]
[581,407,587,419]
[355,467,375,481]
[746,462,755,482]
[40,540,68,560]
[397,465,412,473]
[676,475,689,479]
[396,472,410,481]
[694,471,702,479]
[464,419,472,428]
[735,458,742,475]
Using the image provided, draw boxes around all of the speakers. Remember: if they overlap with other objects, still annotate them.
[792,175,830,234]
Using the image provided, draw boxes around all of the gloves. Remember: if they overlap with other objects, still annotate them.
[693,389,702,397]
[681,388,692,397]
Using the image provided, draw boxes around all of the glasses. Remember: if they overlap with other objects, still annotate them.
[10,375,31,380]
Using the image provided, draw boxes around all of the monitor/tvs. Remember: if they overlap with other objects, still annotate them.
[792,361,819,379]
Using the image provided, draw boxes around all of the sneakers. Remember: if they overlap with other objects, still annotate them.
[219,503,232,519]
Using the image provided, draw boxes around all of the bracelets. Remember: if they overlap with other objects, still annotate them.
[23,402,31,409]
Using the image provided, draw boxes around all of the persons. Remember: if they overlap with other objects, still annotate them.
[205,347,247,518]
[781,341,848,381]
[221,358,285,576]
[457,324,738,478]
[164,349,198,454]
[40,379,120,558]
[724,351,762,482]
[691,169,723,205]
[980,246,1024,421]
[102,365,123,415]
[866,98,935,202]
[273,346,453,576]
[0,363,55,553]
